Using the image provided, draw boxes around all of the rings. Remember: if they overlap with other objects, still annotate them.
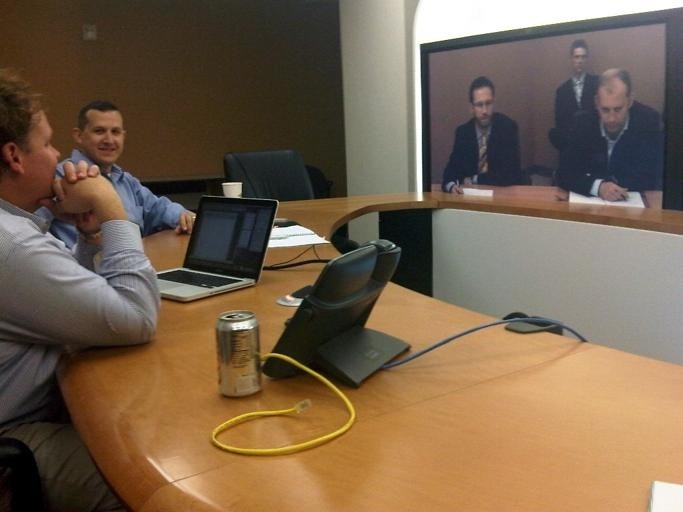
[191,216,196,219]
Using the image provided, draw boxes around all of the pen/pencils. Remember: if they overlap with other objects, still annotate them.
[456,179,460,187]
[611,175,627,201]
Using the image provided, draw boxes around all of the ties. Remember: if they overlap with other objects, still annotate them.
[576,81,582,106]
[479,128,488,175]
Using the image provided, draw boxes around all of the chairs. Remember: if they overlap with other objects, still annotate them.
[222,148,361,257]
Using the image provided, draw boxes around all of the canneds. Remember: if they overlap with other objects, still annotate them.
[215,310,263,398]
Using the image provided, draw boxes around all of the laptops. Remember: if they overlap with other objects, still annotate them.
[154,194,280,303]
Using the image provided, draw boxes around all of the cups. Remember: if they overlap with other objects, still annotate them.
[221,181,243,198]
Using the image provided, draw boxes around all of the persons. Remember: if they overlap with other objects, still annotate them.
[33,101,197,267]
[440,76,521,195]
[553,38,601,186]
[0,69,162,511]
[553,68,664,202]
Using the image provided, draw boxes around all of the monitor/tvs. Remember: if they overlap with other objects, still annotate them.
[262,238,400,388]
